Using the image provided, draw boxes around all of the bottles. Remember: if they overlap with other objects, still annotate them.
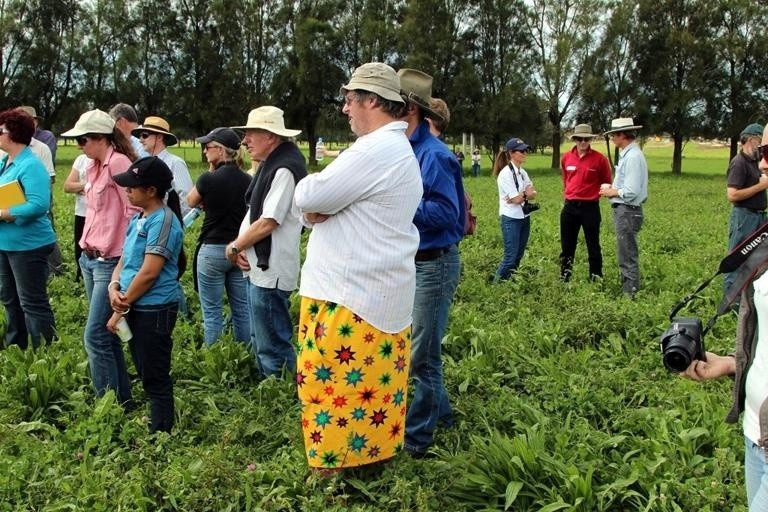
[315,137,325,160]
[182,207,201,229]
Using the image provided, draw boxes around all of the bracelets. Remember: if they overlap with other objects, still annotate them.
[108,280,119,291]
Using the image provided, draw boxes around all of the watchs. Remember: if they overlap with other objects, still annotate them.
[232,242,238,254]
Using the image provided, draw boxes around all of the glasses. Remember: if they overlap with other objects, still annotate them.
[0,128,10,135]
[576,137,592,142]
[512,149,527,153]
[757,144,768,163]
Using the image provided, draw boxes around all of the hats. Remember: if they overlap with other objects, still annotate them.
[61,109,179,188]
[506,138,530,151]
[743,123,764,135]
[195,106,302,150]
[16,106,44,120]
[338,61,444,122]
[572,124,598,137]
[604,118,643,135]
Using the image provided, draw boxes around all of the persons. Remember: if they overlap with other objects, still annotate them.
[492,138,537,284]
[559,123,613,282]
[599,117,649,293]
[61,109,143,414]
[394,66,466,453]
[471,149,481,176]
[187,127,251,347]
[106,155,186,437]
[724,123,768,306]
[0,107,64,349]
[454,147,465,172]
[226,107,307,381]
[677,123,768,512]
[64,105,193,280]
[293,60,424,470]
[315,96,451,157]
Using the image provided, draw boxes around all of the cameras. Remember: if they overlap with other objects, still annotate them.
[523,200,540,214]
[659,316,706,372]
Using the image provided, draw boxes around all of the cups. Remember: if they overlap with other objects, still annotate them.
[112,316,133,343]
[600,183,613,190]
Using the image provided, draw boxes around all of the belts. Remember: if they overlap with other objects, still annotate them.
[84,249,116,261]
[416,247,450,261]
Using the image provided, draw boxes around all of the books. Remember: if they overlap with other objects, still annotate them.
[1,180,27,223]
